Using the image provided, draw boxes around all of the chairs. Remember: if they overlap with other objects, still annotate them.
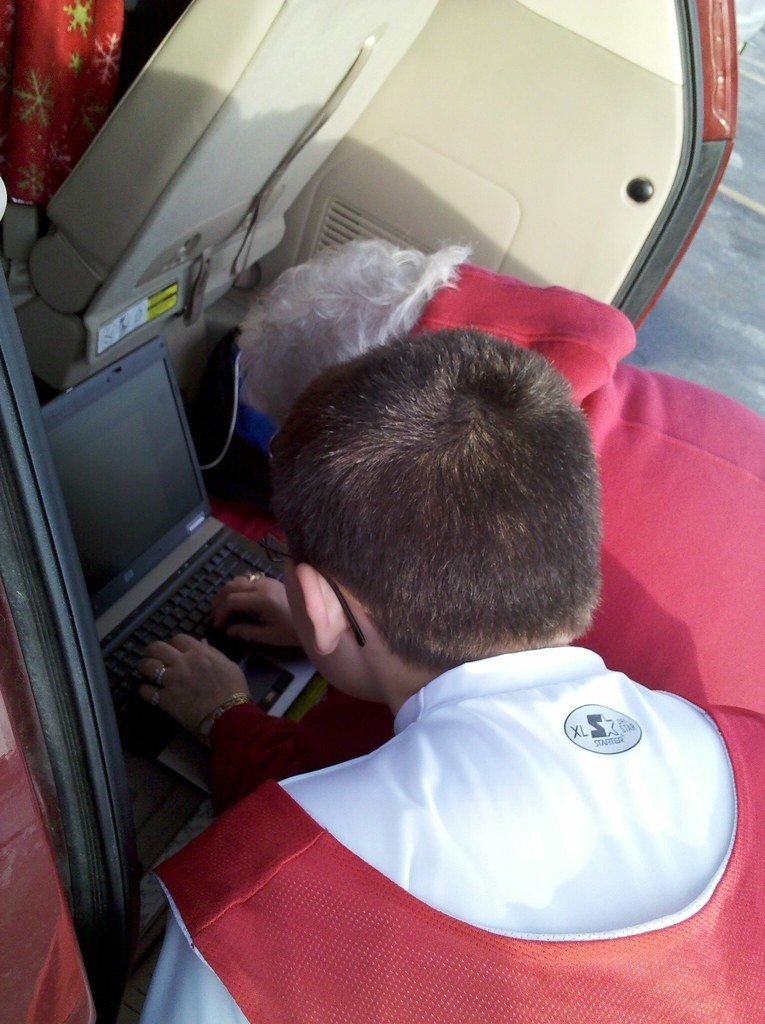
[0,0,439,388]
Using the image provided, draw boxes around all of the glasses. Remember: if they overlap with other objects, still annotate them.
[258,526,365,646]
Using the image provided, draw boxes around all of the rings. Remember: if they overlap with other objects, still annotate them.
[157,667,165,685]
[249,571,265,582]
[149,691,159,705]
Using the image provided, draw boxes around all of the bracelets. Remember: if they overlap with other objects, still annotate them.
[197,693,253,747]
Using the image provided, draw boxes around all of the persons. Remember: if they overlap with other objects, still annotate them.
[136,325,765,1024]
[139,234,765,795]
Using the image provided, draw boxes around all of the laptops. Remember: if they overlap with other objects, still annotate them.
[38,334,319,795]
